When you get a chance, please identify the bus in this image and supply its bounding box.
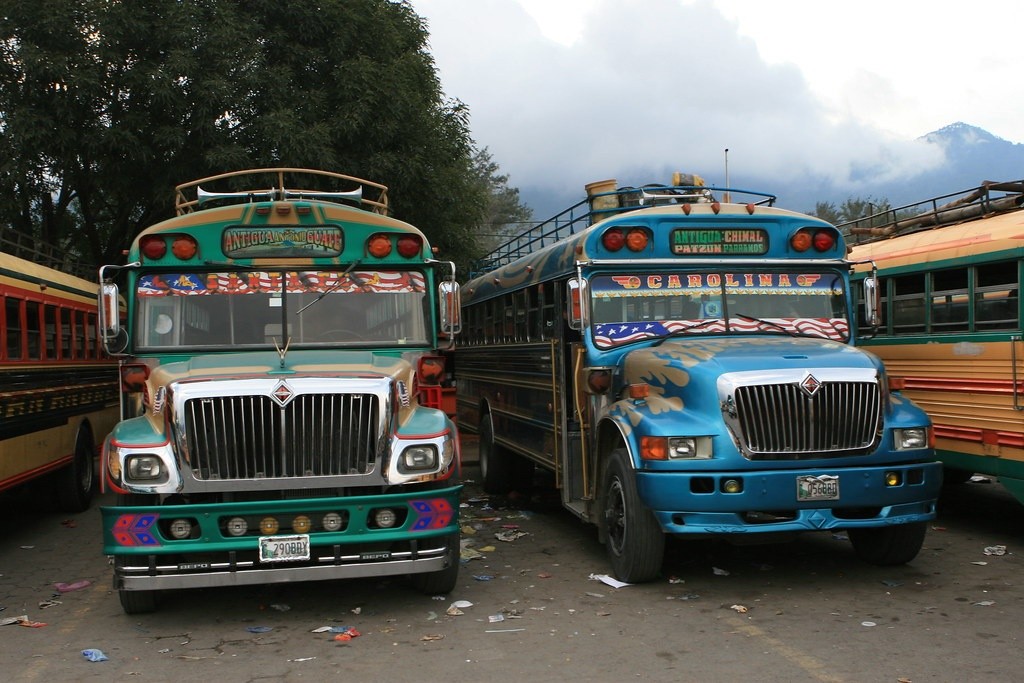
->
[93,167,463,615]
[452,171,950,583]
[0,224,131,516]
[829,179,1024,490]
